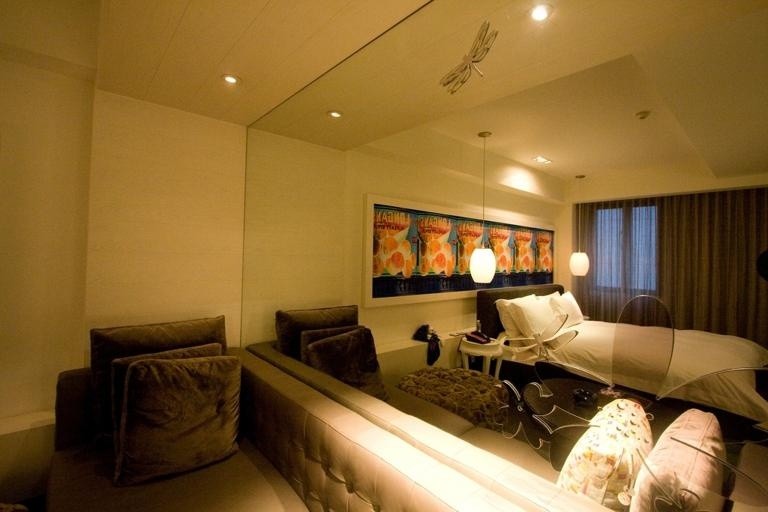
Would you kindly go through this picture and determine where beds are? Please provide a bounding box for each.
[477,284,768,435]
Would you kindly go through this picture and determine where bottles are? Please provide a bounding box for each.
[476,320,481,332]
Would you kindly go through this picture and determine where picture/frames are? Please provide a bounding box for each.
[362,193,557,308]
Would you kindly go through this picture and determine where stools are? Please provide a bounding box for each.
[459,337,503,380]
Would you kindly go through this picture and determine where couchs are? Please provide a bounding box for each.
[246,340,768,512]
[47,348,526,511]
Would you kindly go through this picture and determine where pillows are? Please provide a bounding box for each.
[112,344,221,459]
[116,357,241,483]
[553,399,653,512]
[537,290,560,309]
[507,294,556,337]
[550,292,584,326]
[90,315,224,452]
[626,408,726,511]
[300,325,366,366]
[493,294,537,336]
[275,305,359,363]
[308,328,389,402]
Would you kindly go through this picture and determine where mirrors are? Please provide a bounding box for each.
[240,1,767,512]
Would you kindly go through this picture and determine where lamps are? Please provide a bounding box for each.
[568,175,590,276]
[470,132,495,283]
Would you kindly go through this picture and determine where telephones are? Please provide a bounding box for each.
[465,331,490,343]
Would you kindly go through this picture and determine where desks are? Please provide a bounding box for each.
[524,378,652,472]
[396,367,510,432]
